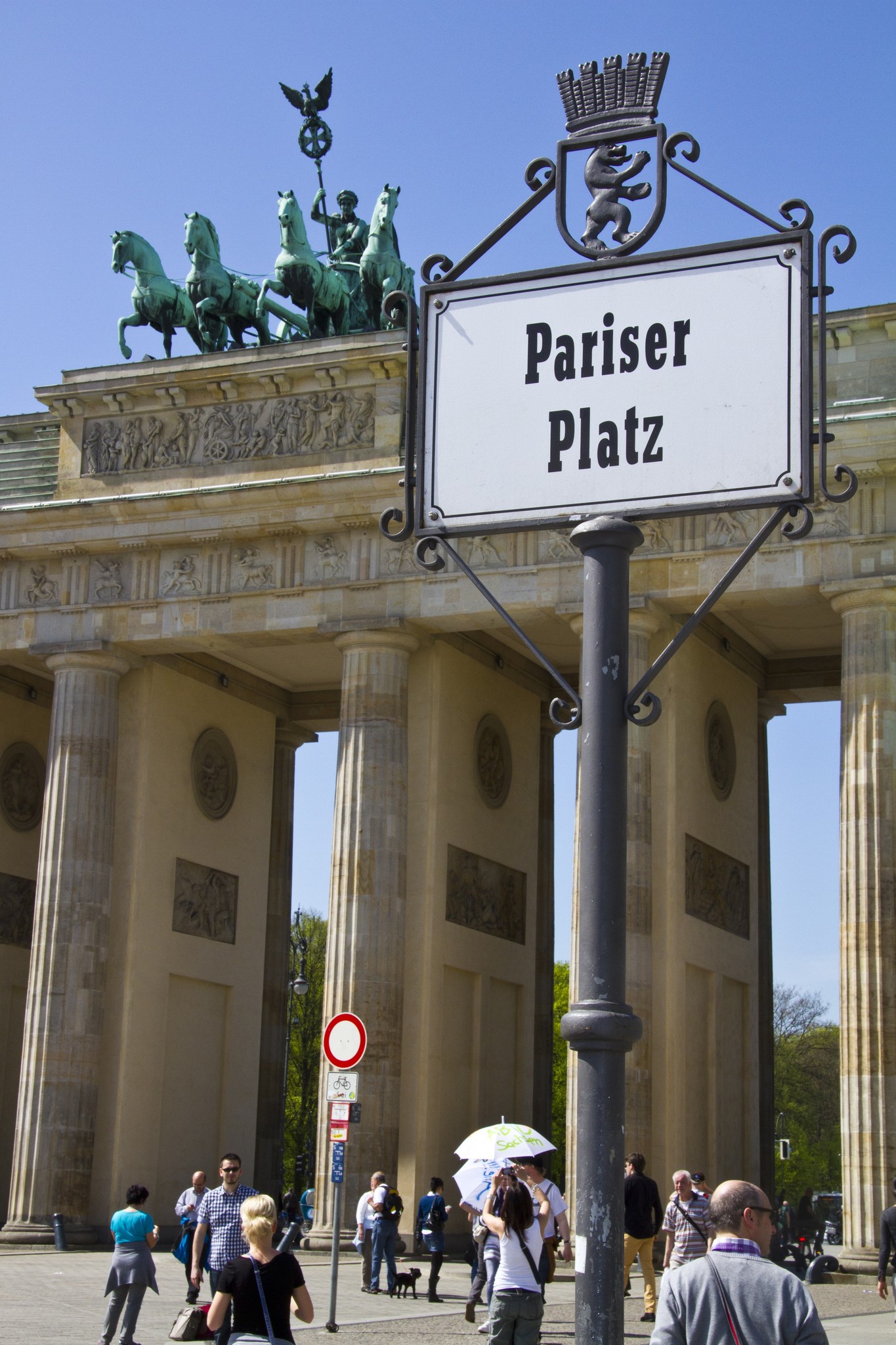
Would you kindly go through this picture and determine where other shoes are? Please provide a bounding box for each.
[624,1292,631,1298]
[186,1296,196,1304]
[476,1298,483,1304]
[118,1340,142,1345]
[465,1302,475,1323]
[97,1339,110,1345]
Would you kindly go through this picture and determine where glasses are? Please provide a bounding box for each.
[624,1164,630,1168]
[741,1206,776,1226]
[222,1167,240,1172]
[441,1187,444,1191]
[194,1182,205,1186]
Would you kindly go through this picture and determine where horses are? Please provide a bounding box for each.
[108,229,196,358]
[259,189,353,338]
[174,212,259,355]
[361,182,416,330]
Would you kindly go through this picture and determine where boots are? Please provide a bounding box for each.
[428,1275,444,1303]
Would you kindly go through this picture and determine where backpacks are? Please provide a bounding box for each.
[374,1186,404,1221]
[169,1306,207,1341]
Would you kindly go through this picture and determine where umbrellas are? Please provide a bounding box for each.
[453,1116,558,1192]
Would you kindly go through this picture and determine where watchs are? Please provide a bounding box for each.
[153,1233,159,1240]
[564,1240,572,1245]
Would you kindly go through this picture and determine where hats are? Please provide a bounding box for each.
[691,1171,705,1182]
[515,1154,542,1164]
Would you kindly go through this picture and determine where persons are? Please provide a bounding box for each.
[282,1188,299,1230]
[204,1195,315,1345]
[616,1149,824,1327]
[175,1170,213,1304]
[190,1152,265,1345]
[503,1156,573,1345]
[650,1180,829,1345]
[413,1175,451,1304]
[310,187,368,326]
[478,1170,522,1335]
[481,1164,550,1345]
[876,1178,896,1321]
[97,1185,160,1345]
[464,1180,571,1324]
[352,1171,404,1298]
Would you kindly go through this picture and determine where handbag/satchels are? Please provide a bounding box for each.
[463,1225,489,1268]
[193,1303,217,1338]
[425,1195,445,1232]
[538,1243,550,1305]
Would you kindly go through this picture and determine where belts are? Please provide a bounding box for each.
[373,1216,388,1223]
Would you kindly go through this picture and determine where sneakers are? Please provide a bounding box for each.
[367,1289,378,1294]
[361,1287,383,1293]
[477,1319,489,1334]
[640,1312,656,1322]
[381,1289,397,1295]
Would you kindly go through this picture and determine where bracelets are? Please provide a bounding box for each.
[531,1183,539,1192]
[485,1193,495,1201]
